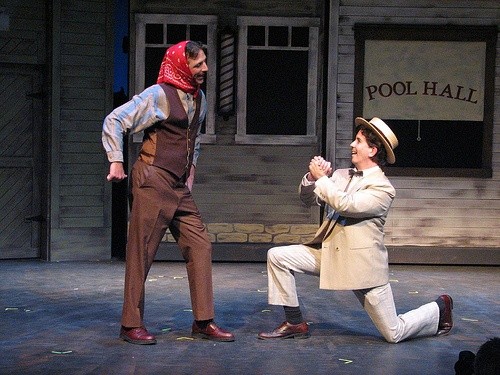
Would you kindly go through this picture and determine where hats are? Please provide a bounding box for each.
[355,117,398,164]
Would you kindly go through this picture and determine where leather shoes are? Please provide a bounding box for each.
[257,321,310,340]
[120,325,155,345]
[435,294,453,337]
[191,321,235,341]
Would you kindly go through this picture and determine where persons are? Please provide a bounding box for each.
[471,337,500,375]
[101,40,235,344]
[258,118,454,343]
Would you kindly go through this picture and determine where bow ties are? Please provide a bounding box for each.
[349,169,363,176]
